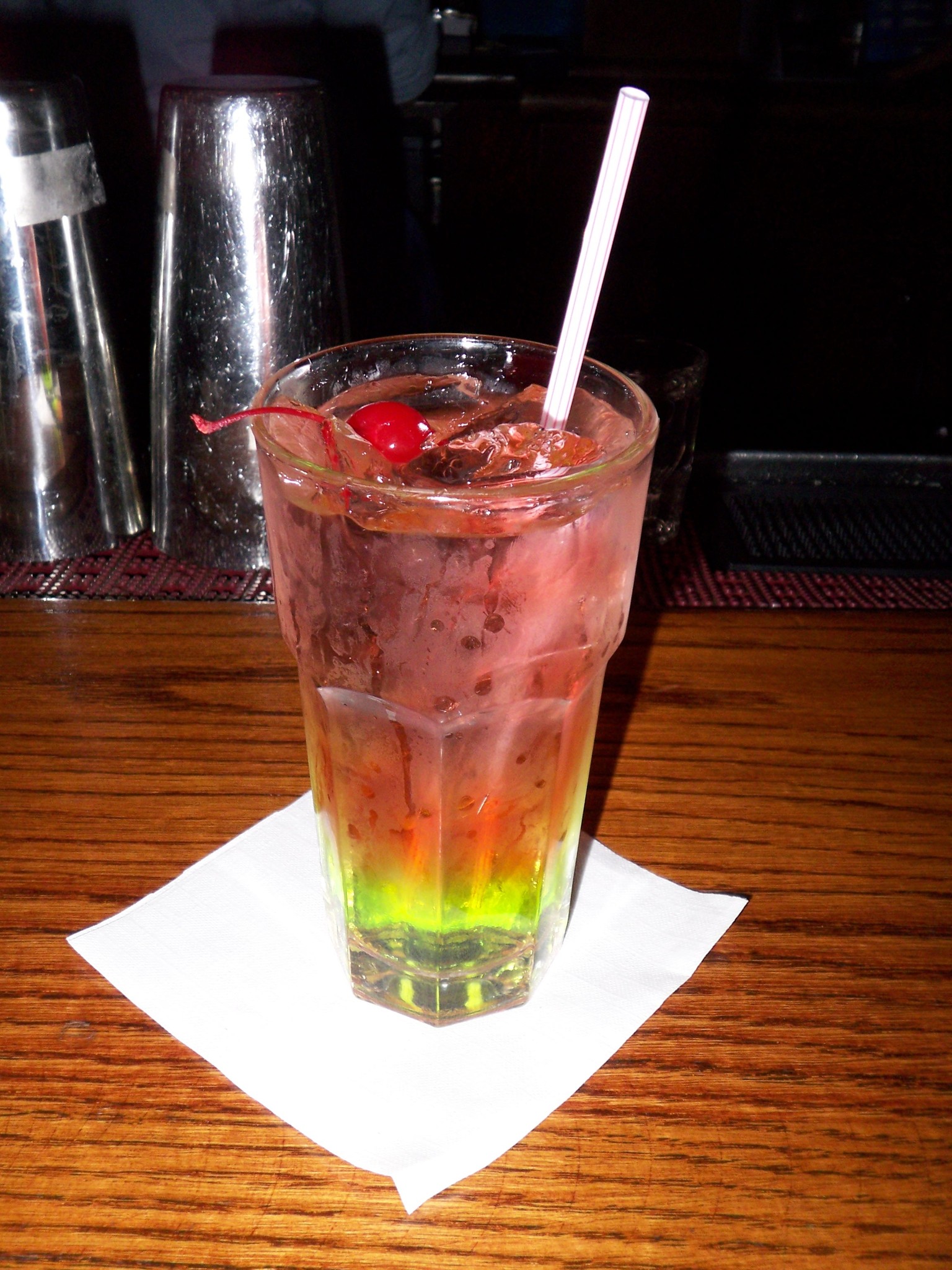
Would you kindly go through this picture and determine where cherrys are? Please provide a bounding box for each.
[192,403,432,468]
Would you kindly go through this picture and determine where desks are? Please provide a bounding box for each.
[0,452,952,1270]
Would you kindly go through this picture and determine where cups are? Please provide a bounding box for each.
[152,72,347,576]
[0,77,146,561]
[247,334,661,1029]
